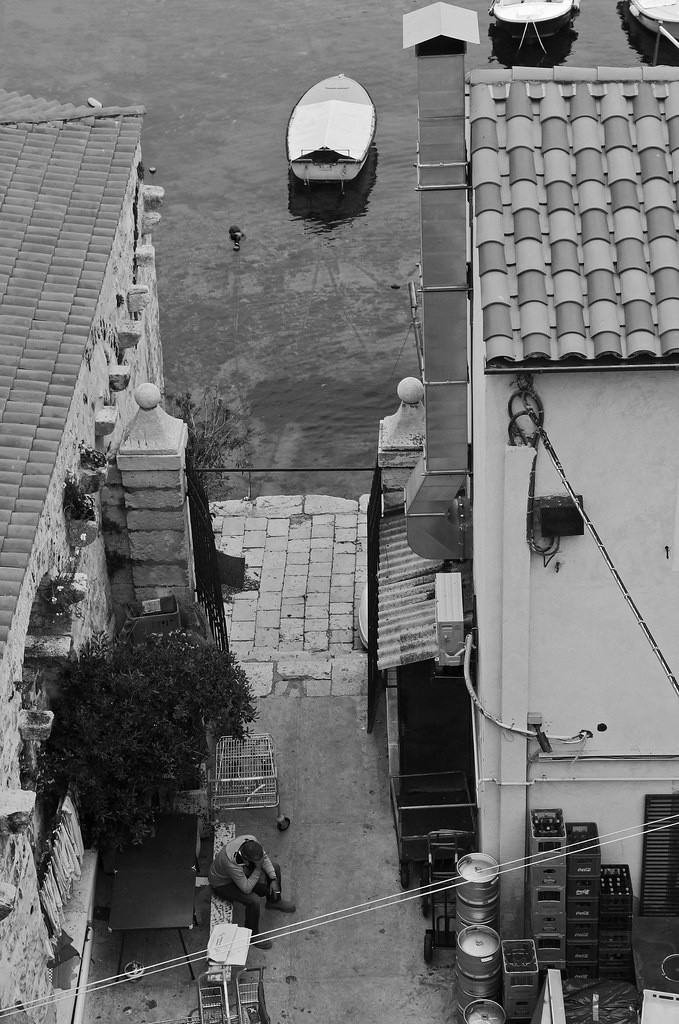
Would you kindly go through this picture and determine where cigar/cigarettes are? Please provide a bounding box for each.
[276,892,280,893]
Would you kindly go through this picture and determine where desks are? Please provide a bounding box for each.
[107,813,199,932]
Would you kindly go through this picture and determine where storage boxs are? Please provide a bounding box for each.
[124,593,181,646]
[501,808,634,1024]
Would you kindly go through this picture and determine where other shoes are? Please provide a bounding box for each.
[251,933,272,949]
[266,900,296,912]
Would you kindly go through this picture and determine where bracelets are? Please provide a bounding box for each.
[271,878,276,881]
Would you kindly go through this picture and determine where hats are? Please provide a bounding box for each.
[242,842,264,863]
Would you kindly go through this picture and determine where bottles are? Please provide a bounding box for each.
[568,830,598,854]
[600,867,630,904]
[532,809,565,852]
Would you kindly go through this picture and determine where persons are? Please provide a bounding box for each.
[208,835,296,949]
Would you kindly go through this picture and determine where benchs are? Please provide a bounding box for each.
[206,822,236,982]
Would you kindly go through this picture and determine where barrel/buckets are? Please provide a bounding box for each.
[456,852,507,1024]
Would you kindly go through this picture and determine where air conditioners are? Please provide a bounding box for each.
[435,572,466,668]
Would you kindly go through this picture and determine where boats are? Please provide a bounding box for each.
[488,0,581,39]
[285,72,378,196]
[286,137,379,232]
[629,0,679,42]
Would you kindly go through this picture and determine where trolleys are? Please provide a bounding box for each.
[209,732,290,832]
